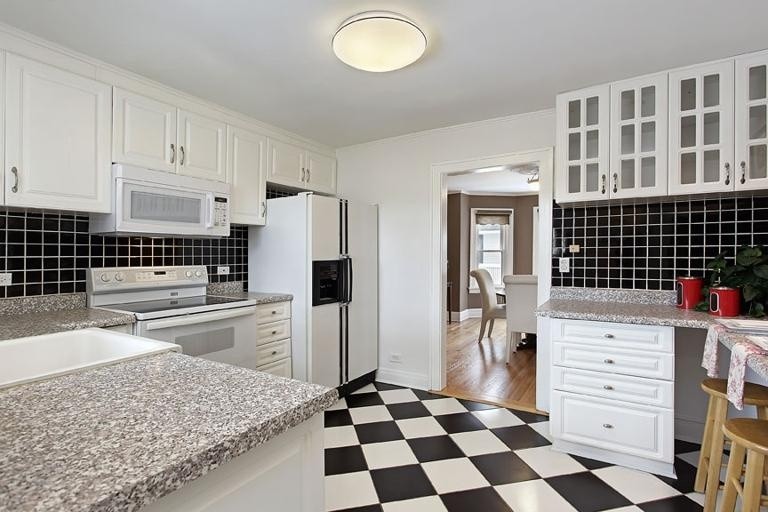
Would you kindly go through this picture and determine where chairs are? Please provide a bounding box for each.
[503,274,538,366]
[470,268,520,344]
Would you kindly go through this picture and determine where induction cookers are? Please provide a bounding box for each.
[94,293,249,314]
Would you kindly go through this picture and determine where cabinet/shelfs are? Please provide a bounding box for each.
[227,127,266,226]
[258,302,293,381]
[267,138,337,193]
[0,51,111,216]
[668,50,768,196]
[550,319,675,466]
[556,73,667,204]
[111,90,233,184]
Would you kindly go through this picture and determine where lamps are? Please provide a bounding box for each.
[332,11,427,74]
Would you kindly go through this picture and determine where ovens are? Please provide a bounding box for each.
[142,321,257,357]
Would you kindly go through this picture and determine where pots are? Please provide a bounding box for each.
[675,275,705,309]
[709,287,740,317]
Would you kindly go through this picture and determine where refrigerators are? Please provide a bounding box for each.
[306,192,378,380]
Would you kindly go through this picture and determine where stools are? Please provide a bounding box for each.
[694,378,768,512]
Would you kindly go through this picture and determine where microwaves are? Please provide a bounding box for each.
[115,179,232,238]
